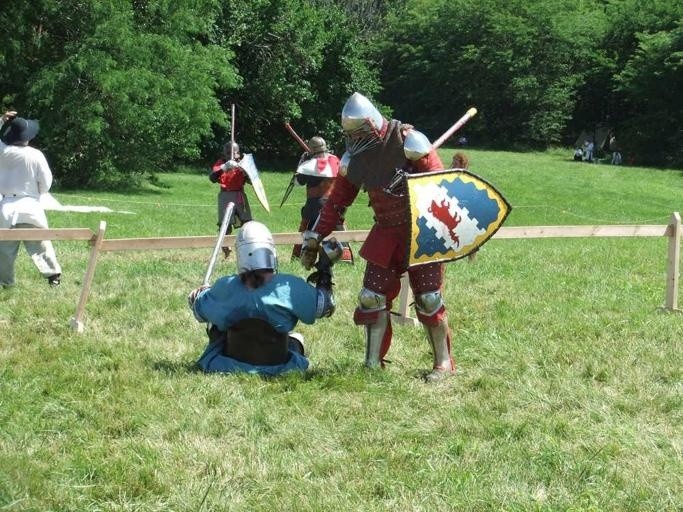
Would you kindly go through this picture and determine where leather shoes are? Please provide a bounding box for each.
[301,152,309,159]
[300,231,322,271]
[314,239,344,271]
[220,160,237,172]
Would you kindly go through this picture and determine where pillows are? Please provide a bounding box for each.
[222,141,240,161]
[309,136,329,154]
[235,221,278,275]
[341,92,383,157]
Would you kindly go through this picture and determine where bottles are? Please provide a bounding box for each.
[0,118,39,145]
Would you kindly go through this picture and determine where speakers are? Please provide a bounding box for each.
[415,299,455,381]
[354,303,392,368]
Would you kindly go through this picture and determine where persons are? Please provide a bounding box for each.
[304,92,458,385]
[573,128,623,165]
[209,139,259,260]
[188,219,333,377]
[293,137,352,267]
[0,109,65,293]
[438,151,488,265]
